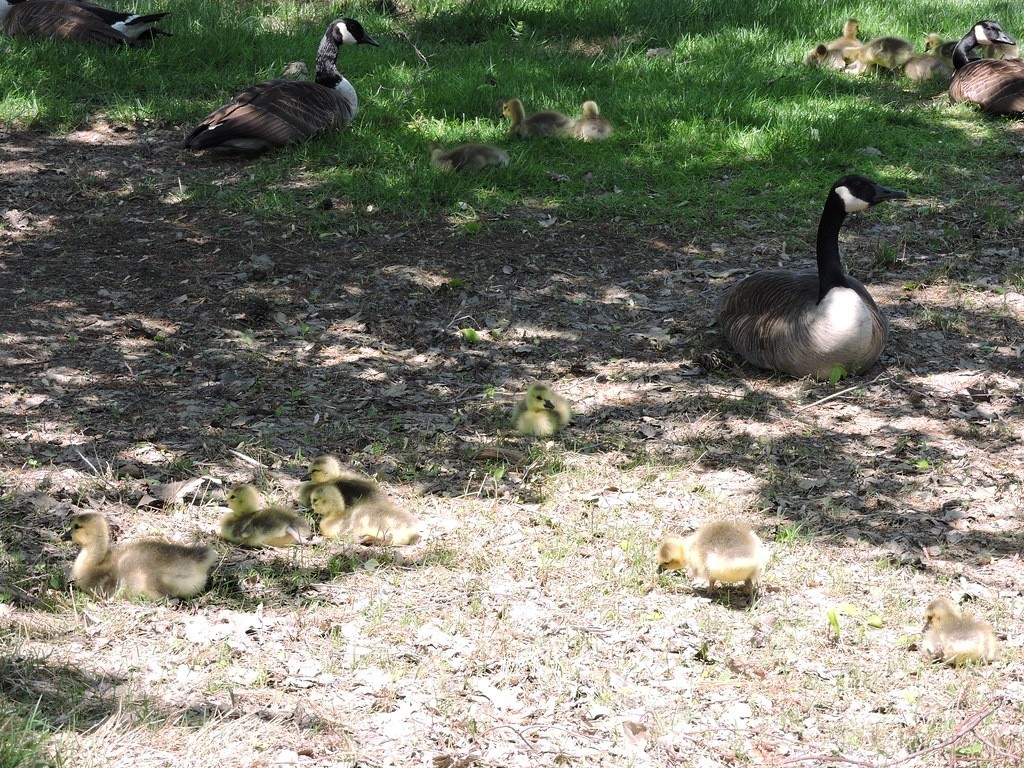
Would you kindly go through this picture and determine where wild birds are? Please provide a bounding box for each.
[218,484,324,548]
[428,98,614,177]
[0,0,175,52]
[655,519,769,599]
[60,509,217,603]
[919,597,999,666]
[179,17,381,156]
[510,385,572,438]
[716,173,909,383]
[299,456,429,546]
[801,19,1024,116]
